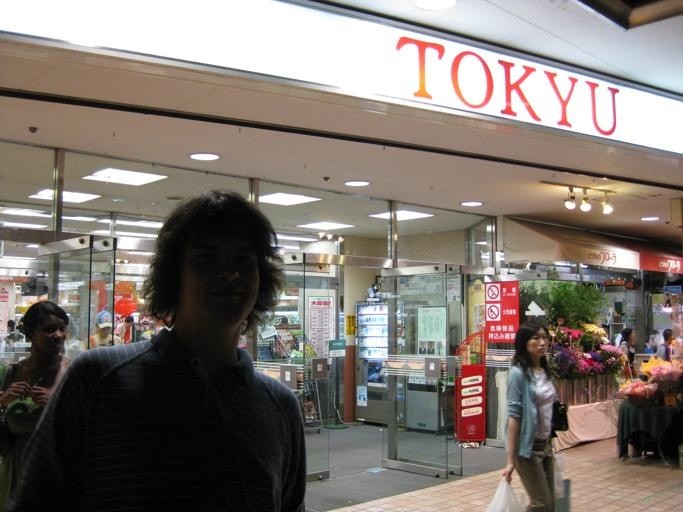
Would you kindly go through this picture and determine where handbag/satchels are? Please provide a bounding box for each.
[553,401,568,430]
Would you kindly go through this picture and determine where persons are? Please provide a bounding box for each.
[114,315,134,344]
[13,190,309,511]
[84,310,124,352]
[618,327,639,380]
[274,315,299,359]
[4,318,24,346]
[656,328,673,364]
[0,299,76,511]
[499,318,563,512]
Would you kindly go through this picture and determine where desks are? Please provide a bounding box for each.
[617,401,682,458]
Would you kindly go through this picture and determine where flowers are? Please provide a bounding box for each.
[546,319,682,400]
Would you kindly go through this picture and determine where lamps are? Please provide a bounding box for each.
[563,185,592,212]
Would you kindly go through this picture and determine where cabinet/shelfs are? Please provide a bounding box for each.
[354,299,428,428]
